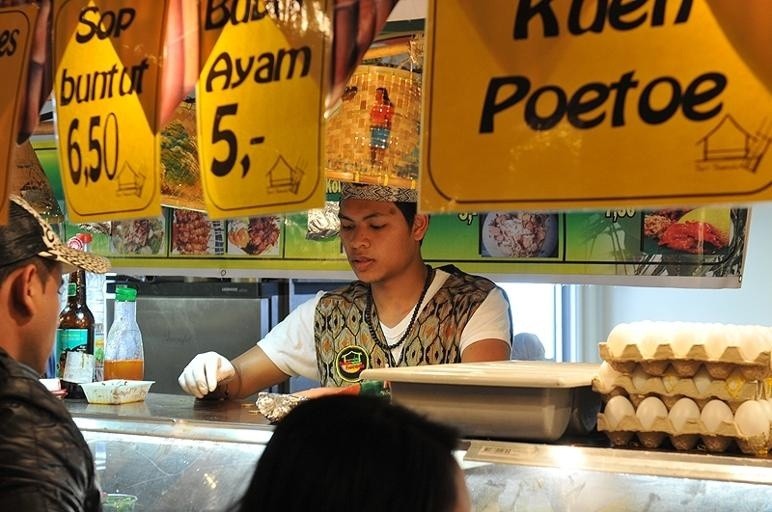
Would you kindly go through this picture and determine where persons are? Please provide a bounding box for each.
[0,190,111,512]
[223,394,472,512]
[176,180,512,403]
[370,88,396,166]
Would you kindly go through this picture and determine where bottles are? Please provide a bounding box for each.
[56,232,145,400]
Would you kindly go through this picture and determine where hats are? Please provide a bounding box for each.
[0,193,111,274]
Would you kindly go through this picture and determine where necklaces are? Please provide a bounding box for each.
[365,264,432,349]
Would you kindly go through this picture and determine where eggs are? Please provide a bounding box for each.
[597,322,772,434]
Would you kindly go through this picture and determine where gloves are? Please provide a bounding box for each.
[177,351,236,399]
